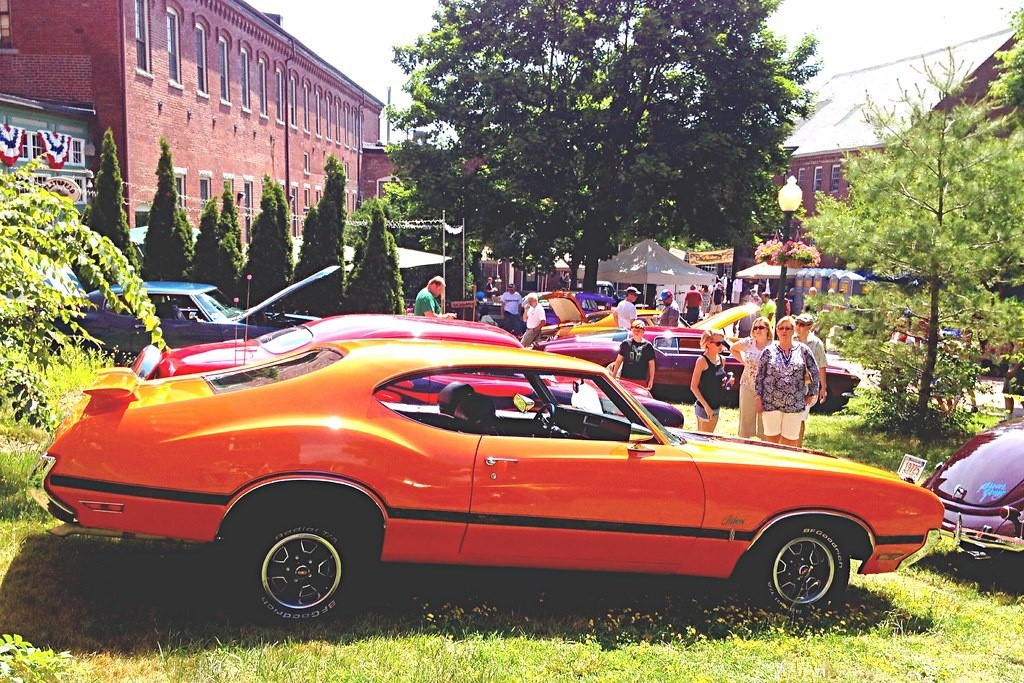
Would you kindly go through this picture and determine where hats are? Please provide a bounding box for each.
[632,320,645,329]
[507,283,515,289]
[750,289,756,292]
[627,286,641,294]
[797,313,813,322]
[658,289,672,300]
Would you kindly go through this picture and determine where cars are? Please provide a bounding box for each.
[920,415,1024,595]
[548,303,762,339]
[132,312,655,414]
[476,281,625,333]
[534,326,861,417]
[74,265,347,368]
[27,338,946,623]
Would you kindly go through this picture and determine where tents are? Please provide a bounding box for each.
[569,238,717,310]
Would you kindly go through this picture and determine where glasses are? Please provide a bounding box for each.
[796,321,811,327]
[709,341,724,345]
[754,326,767,330]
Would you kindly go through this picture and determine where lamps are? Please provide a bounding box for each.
[84,145,96,157]
[289,195,295,201]
[86,178,93,188]
[238,192,246,200]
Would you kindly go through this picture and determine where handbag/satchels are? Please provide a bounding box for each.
[711,304,717,312]
[801,346,815,404]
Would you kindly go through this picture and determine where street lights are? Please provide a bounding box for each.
[777,174,803,325]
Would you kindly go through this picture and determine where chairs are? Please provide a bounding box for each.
[452,392,503,432]
[438,381,480,413]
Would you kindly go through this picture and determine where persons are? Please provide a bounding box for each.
[699,273,731,318]
[690,329,735,433]
[479,307,498,327]
[889,309,1024,423]
[614,287,641,331]
[520,291,546,347]
[801,287,856,331]
[414,276,456,319]
[500,283,523,337]
[681,285,702,327]
[753,316,819,446]
[733,281,793,339]
[792,313,828,449]
[653,289,680,327]
[730,317,774,441]
[556,271,569,291]
[484,276,498,302]
[613,319,656,391]
